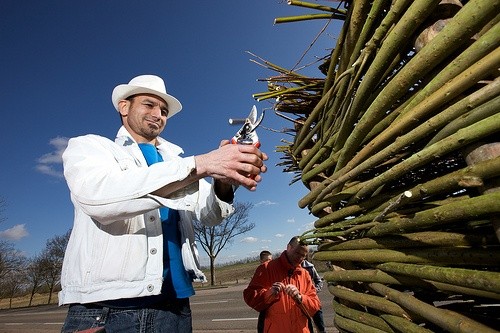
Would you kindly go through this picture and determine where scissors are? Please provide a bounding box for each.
[227,105,265,149]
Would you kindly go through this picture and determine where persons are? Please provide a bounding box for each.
[298,255,327,333]
[259,250,272,264]
[242,236,322,333]
[57,75,268,333]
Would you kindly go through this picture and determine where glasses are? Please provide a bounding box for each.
[288,242,306,260]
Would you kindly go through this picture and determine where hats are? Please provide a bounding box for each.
[111,74,183,120]
